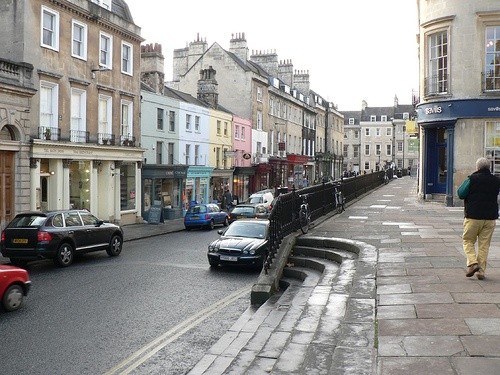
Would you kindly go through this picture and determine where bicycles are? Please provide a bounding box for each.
[298,193,314,234]
[382,166,389,185]
[331,182,346,214]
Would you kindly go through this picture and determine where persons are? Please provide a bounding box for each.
[225,190,233,211]
[457,157,500,280]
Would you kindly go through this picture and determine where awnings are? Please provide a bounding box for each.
[142,164,189,179]
[287,154,309,164]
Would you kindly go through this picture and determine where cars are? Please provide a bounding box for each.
[184,193,276,231]
[207,217,271,271]
[0,265,32,311]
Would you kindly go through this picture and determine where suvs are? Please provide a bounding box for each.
[1,209,124,267]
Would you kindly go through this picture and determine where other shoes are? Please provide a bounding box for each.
[466,263,485,280]
